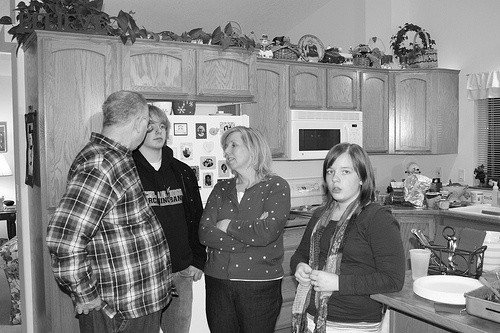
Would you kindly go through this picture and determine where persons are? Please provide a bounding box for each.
[205,175,211,186]
[133,102,208,333]
[289,142,406,333]
[220,163,227,174]
[203,159,213,167]
[45,90,179,333]
[197,127,204,137]
[197,127,291,333]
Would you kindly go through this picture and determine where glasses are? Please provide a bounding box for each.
[141,117,154,132]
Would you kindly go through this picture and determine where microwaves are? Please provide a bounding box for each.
[285,110,363,160]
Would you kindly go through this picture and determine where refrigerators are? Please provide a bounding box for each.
[159,115,249,333]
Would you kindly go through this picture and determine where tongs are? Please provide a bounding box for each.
[410,228,449,275]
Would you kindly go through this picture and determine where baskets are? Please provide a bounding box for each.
[222,20,242,46]
[271,44,309,62]
[380,54,392,66]
[354,57,371,67]
[406,30,438,68]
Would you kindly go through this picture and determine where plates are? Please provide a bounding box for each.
[298,34,325,62]
[413,275,484,305]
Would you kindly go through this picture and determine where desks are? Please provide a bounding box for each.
[0,204,17,239]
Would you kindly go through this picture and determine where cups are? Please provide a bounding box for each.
[471,192,484,203]
[439,199,449,210]
[409,249,431,281]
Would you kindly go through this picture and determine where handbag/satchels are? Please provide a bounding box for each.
[0,235,21,324]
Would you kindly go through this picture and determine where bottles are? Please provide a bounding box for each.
[492,182,498,207]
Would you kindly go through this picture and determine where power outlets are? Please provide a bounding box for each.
[436,167,443,179]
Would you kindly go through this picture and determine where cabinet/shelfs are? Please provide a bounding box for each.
[22,29,500,333]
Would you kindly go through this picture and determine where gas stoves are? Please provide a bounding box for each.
[284,176,326,226]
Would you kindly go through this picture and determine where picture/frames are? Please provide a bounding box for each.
[0,121,8,152]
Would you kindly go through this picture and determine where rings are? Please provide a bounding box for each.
[313,281,315,287]
